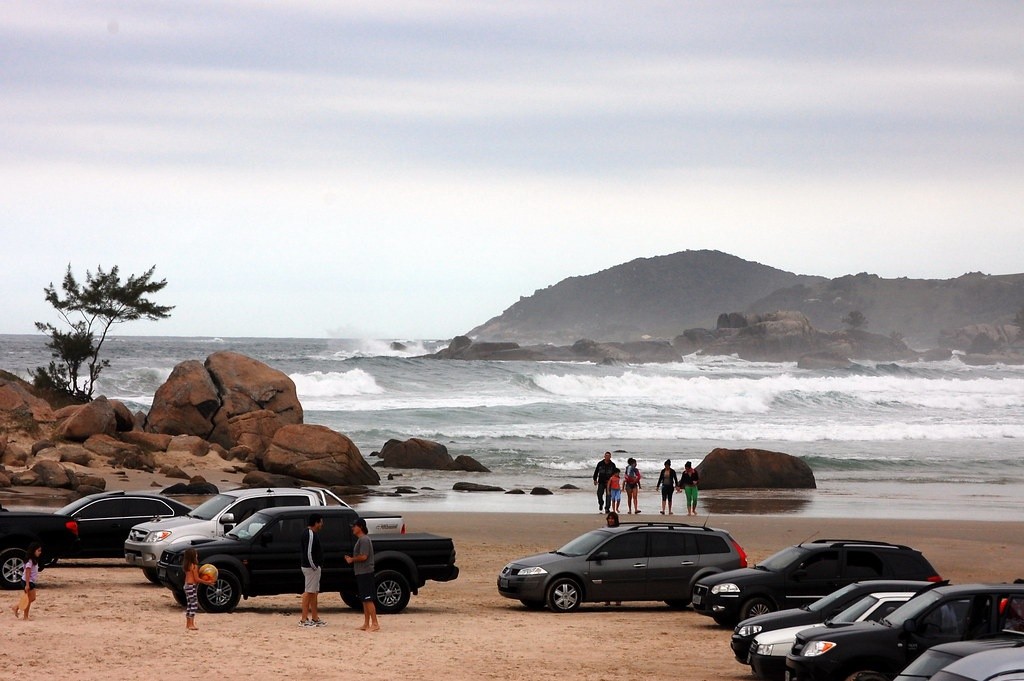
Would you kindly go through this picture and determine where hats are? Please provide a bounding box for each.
[349,518,366,526]
[664,459,671,466]
[685,461,691,467]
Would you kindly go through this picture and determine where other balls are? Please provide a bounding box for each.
[199,564,219,584]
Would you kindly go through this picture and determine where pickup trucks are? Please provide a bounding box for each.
[156,506,459,614]
[125,487,406,588]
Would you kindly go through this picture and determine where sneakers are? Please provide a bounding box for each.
[311,618,328,626]
[298,619,314,628]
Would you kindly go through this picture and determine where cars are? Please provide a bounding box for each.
[731,580,1024,681]
[0,503,80,590]
[43,491,194,568]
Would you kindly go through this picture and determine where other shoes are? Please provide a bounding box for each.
[604,601,610,608]
[660,511,665,515]
[635,510,641,514]
[599,501,604,511]
[616,601,621,607]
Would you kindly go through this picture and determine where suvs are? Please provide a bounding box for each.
[497,521,748,613]
[693,538,943,628]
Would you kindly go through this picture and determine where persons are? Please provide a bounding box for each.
[656,459,680,515]
[927,574,960,636]
[298,516,328,629]
[679,461,698,516]
[607,468,621,513]
[622,457,643,515]
[603,513,623,606]
[10,546,43,623]
[182,547,215,630]
[345,519,379,633]
[593,453,616,513]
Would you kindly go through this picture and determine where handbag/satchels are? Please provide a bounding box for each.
[19,590,29,610]
